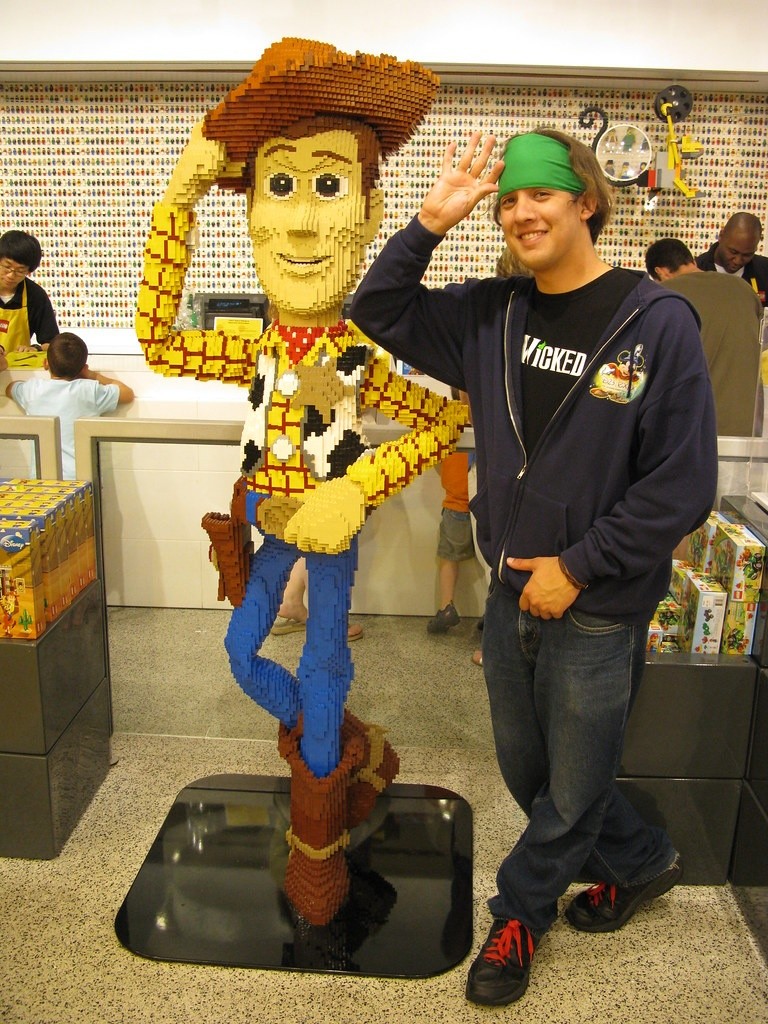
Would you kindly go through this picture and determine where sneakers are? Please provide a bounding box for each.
[465,918,540,1006]
[566,860,684,933]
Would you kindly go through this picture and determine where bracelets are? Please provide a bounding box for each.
[556,556,589,590]
[31,344,43,351]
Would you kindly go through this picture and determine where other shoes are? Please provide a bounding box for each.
[477,616,484,630]
[428,600,459,633]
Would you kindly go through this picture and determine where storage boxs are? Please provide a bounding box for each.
[721,596,758,655]
[649,621,662,653]
[661,641,679,653]
[688,511,730,573]
[0,494,79,600]
[0,521,45,640]
[669,558,699,605]
[655,594,680,634]
[710,524,765,603]
[0,507,59,623]
[0,484,87,592]
[0,500,71,613]
[0,478,97,582]
[677,572,728,655]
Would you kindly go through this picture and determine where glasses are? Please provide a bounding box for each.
[0,263,29,276]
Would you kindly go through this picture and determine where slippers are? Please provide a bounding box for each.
[348,624,363,641]
[271,618,306,635]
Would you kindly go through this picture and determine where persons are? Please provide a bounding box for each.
[428,385,494,666]
[140,32,474,939]
[646,239,765,439]
[270,550,365,645]
[5,332,134,483]
[349,125,717,1008]
[698,212,767,311]
[0,230,60,354]
